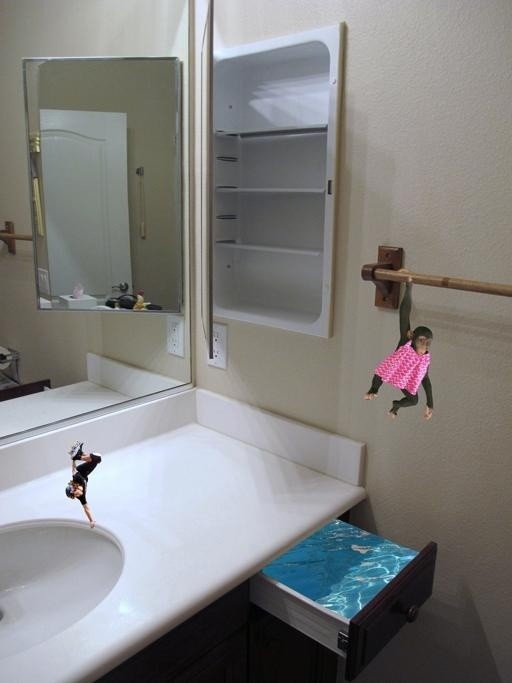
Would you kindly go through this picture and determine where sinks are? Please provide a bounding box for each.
[1,517,125,661]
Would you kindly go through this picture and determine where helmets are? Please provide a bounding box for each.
[66,486,75,499]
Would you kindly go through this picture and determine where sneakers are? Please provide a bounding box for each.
[72,442,84,460]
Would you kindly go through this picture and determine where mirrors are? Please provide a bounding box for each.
[1,56,193,439]
[20,57,185,313]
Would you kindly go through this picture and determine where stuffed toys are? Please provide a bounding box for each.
[362,268,434,420]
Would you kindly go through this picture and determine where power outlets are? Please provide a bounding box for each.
[207,322,228,371]
[165,316,185,359]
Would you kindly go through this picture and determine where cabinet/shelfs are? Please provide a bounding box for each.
[211,21,346,339]
[94,508,438,682]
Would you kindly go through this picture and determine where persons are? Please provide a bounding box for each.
[64,440,103,527]
